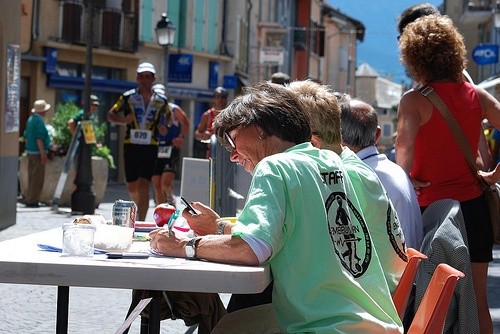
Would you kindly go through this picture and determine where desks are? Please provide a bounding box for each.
[0,220,271,334]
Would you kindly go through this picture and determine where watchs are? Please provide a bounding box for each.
[216,222,227,235]
[186,237,201,261]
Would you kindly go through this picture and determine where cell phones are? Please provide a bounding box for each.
[104,253,150,258]
[181,196,198,215]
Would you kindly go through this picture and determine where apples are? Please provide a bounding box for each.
[153,203,176,226]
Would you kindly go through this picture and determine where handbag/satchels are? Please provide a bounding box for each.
[487,183,500,245]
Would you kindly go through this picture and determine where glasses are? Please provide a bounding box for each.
[224,123,248,149]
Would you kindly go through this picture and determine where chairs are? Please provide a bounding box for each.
[393,248,465,334]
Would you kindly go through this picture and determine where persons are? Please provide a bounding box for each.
[226,78,409,314]
[22,99,51,209]
[107,63,190,221]
[395,4,500,334]
[339,99,424,252]
[148,82,403,334]
[195,87,229,158]
[67,94,100,191]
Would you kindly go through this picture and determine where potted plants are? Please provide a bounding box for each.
[19,100,117,208]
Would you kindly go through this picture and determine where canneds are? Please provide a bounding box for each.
[112,200,137,237]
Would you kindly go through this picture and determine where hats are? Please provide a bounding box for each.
[137,62,156,75]
[152,83,166,95]
[31,99,50,113]
[90,95,100,105]
[267,73,291,85]
[213,87,230,95]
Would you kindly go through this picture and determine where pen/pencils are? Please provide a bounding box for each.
[167,209,180,229]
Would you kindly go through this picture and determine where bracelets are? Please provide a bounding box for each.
[200,132,204,141]
[178,135,184,139]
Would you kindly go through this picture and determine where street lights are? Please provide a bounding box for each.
[153,11,177,98]
